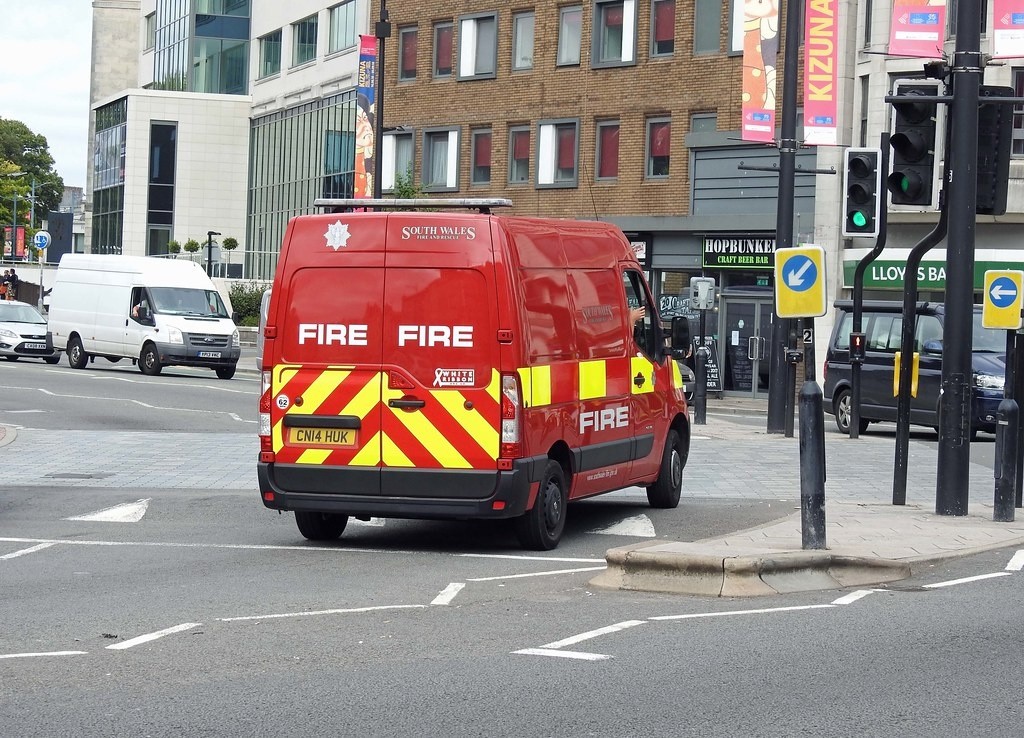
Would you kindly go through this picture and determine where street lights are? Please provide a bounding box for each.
[206,231,221,281]
[0,190,38,265]
[30,179,52,261]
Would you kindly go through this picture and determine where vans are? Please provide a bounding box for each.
[256,289,272,370]
[257,196,693,547]
[823,301,1005,442]
[46,252,241,380]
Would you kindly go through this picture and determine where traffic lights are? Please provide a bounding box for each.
[849,332,867,364]
[841,148,881,238]
[886,80,943,214]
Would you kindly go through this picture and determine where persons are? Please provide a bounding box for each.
[628,306,646,339]
[0,269,18,299]
[132,291,163,318]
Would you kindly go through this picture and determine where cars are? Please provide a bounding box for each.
[43,290,52,312]
[677,361,695,407]
[0,300,62,364]
